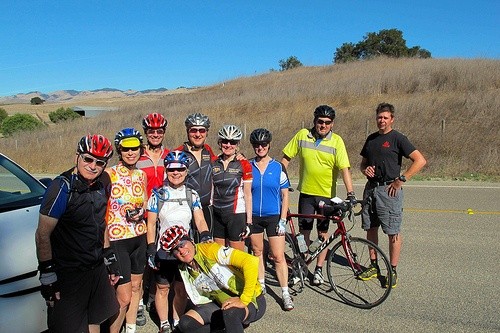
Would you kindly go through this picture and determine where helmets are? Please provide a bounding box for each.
[114,127,143,148]
[314,105,335,122]
[160,225,188,253]
[250,128,272,144]
[217,125,243,144]
[164,150,189,169]
[185,113,210,131]
[77,134,113,161]
[142,112,168,131]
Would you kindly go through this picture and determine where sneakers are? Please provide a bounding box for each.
[385,270,398,288]
[160,323,172,333]
[313,270,324,285]
[136,305,146,325]
[358,267,381,280]
[282,294,293,310]
[262,285,266,294]
[172,322,175,331]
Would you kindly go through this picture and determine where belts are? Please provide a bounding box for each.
[367,180,393,187]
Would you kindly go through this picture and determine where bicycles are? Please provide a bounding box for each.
[248,188,393,309]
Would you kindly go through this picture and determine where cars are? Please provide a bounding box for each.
[0,153,54,333]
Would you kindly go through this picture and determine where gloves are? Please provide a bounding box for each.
[345,196,358,207]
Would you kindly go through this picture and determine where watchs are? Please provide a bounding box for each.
[347,191,355,196]
[399,175,407,182]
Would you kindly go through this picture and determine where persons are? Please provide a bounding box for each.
[359,102,426,288]
[173,112,247,243]
[248,128,295,311]
[146,150,213,333]
[160,225,266,333]
[136,112,171,326]
[210,124,253,252]
[35,134,121,333]
[281,105,357,284]
[103,127,148,333]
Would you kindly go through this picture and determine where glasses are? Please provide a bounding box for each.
[221,139,239,145]
[167,168,186,172]
[119,147,139,152]
[80,154,106,167]
[253,142,268,147]
[147,129,165,134]
[189,128,208,133]
[316,119,332,125]
[172,240,186,254]
